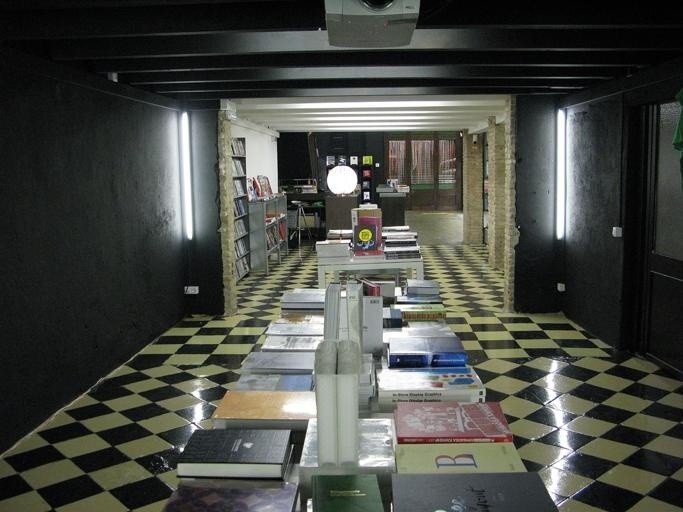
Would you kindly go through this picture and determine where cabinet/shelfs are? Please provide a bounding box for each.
[249,194,288,275]
[231,137,250,283]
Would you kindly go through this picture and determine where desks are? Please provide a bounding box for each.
[377,184,410,226]
[318,255,424,289]
[286,191,361,239]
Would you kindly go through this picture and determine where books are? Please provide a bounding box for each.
[230,140,250,284]
[247,175,274,200]
[375,178,409,193]
[315,202,421,261]
[265,213,287,250]
[176,276,560,511]
